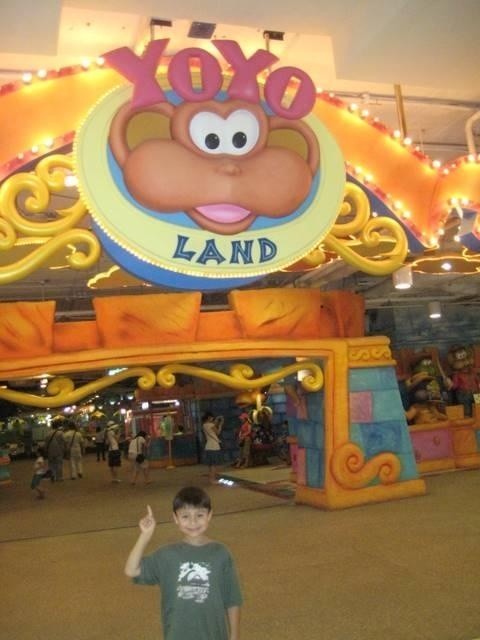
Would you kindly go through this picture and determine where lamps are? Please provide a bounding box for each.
[392,262,414,292]
[424,298,444,320]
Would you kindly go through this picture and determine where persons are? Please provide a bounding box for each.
[0,404,292,499]
[125,486,243,640]
